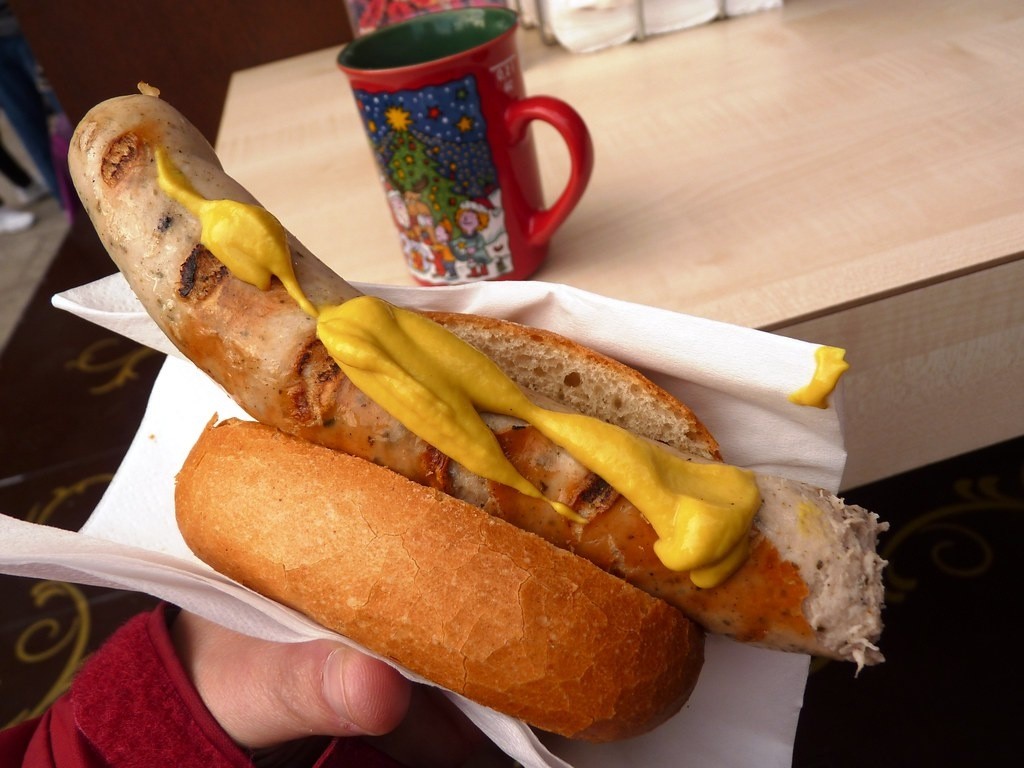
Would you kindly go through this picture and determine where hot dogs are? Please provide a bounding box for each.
[68,94,889,747]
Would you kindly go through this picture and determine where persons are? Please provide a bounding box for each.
[17,605,404,768]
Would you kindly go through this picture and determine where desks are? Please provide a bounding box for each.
[210,3,1024,498]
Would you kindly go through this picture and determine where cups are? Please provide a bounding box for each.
[335,6,594,286]
